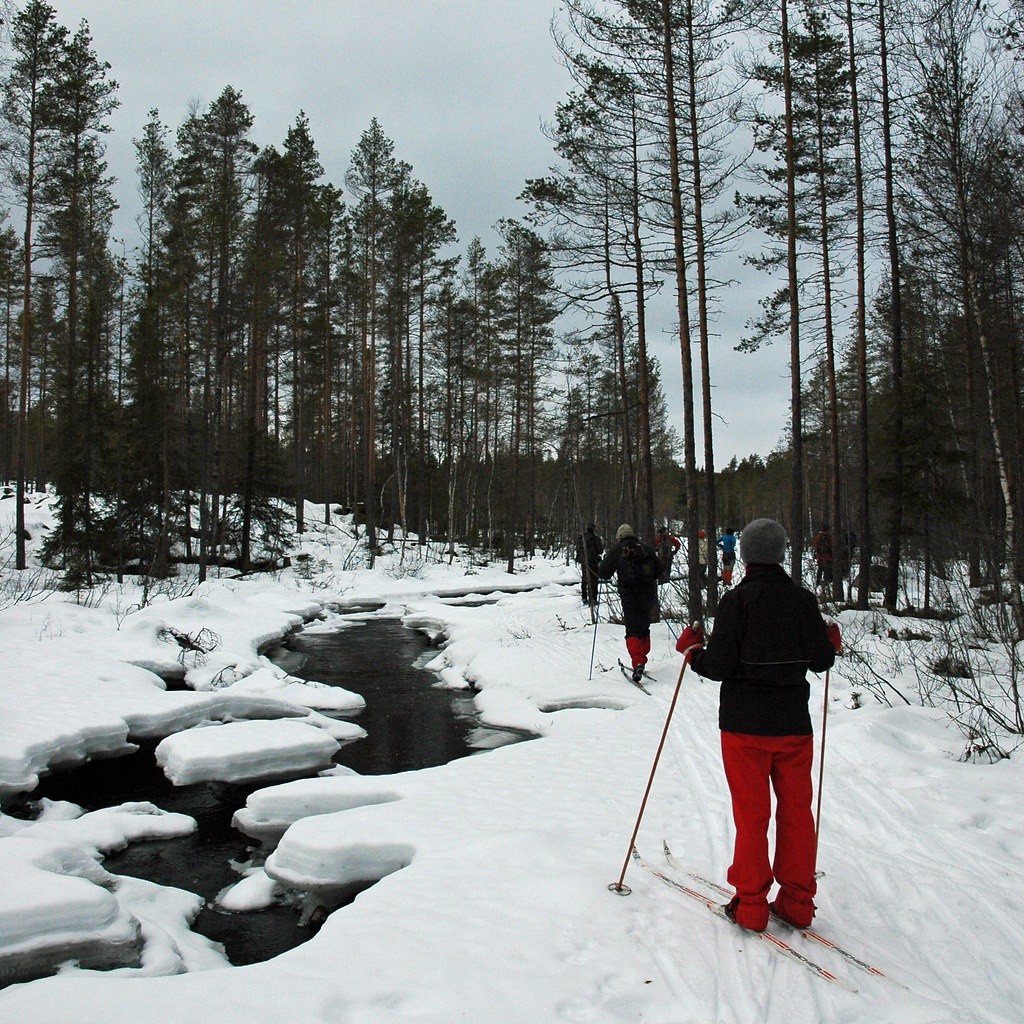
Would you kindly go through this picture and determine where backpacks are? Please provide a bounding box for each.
[659,534,672,559]
[617,541,656,589]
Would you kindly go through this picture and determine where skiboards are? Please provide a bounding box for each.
[618,658,657,696]
[629,839,914,995]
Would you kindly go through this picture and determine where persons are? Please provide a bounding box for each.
[655,526,681,585]
[575,522,603,606]
[698,528,708,588]
[813,525,832,585]
[598,524,663,682]
[716,528,736,586]
[675,518,841,933]
[843,525,857,579]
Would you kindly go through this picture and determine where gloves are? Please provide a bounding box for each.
[676,623,705,656]
[826,624,841,651]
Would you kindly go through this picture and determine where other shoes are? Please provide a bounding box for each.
[633,664,645,682]
[723,582,732,586]
[725,896,740,922]
[769,902,777,915]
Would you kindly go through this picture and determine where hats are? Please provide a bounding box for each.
[698,529,704,537]
[740,518,786,563]
[616,524,633,539]
